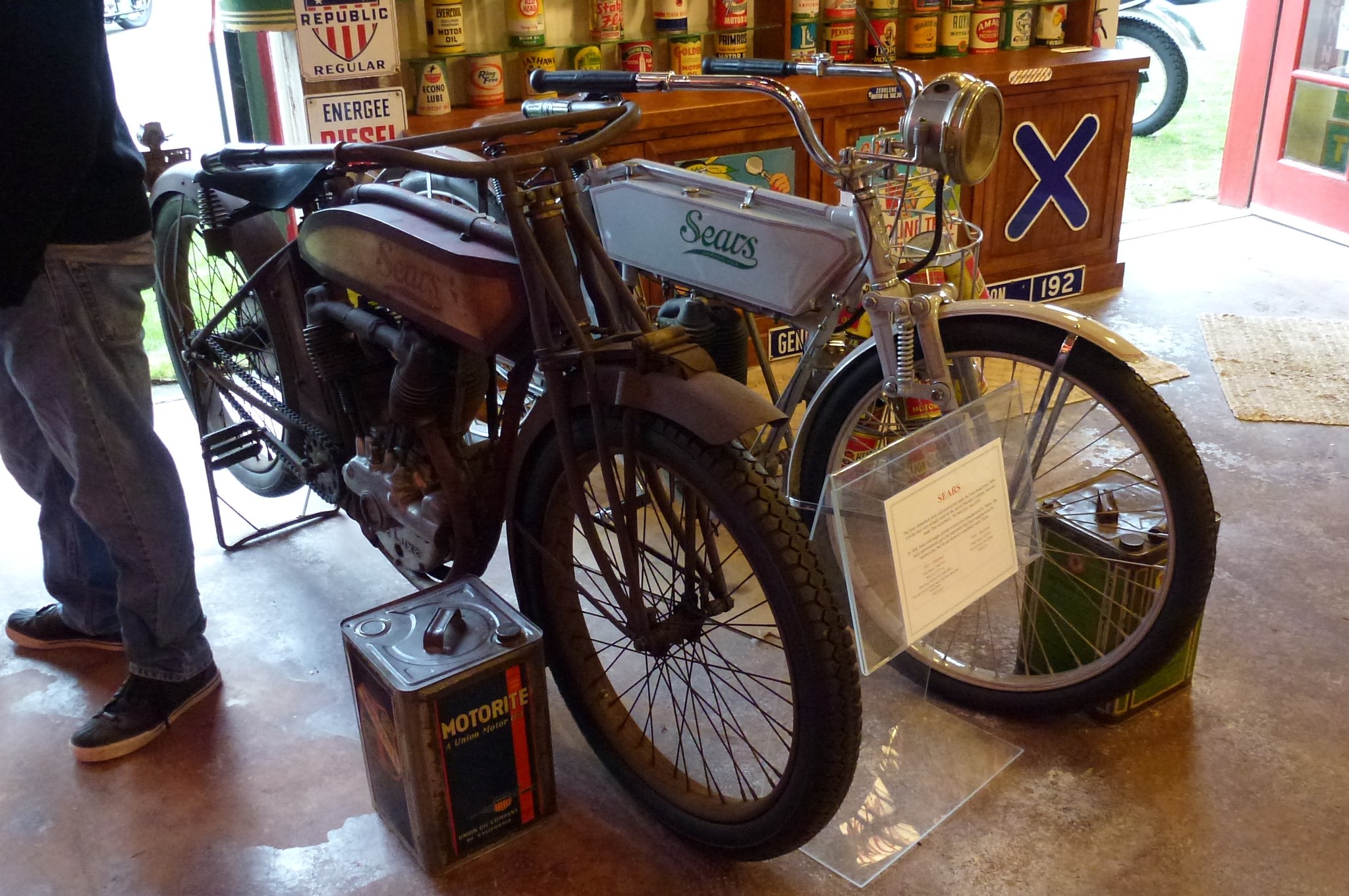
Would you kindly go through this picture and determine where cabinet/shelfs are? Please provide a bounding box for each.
[473,68,1135,416]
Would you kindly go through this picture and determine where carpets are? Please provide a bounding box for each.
[1198,310,1349,429]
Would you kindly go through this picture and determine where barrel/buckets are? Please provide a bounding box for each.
[407,0,747,119]
[1015,468,1221,725]
[340,575,561,882]
[781,0,1072,65]
[820,226,992,499]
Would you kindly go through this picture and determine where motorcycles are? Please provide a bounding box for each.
[1113,0,1207,138]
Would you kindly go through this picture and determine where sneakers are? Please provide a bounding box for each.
[4,602,122,652]
[69,660,222,762]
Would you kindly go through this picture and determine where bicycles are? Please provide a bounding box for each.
[148,51,1223,868]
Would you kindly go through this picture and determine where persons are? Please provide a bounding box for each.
[1,1,225,762]
